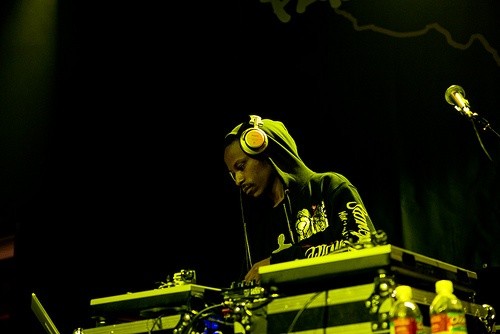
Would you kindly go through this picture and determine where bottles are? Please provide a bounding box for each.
[389,286,423,334]
[430,280,467,334]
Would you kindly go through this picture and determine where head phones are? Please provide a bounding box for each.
[239,114,268,156]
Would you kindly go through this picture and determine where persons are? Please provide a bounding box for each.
[223,115,377,285]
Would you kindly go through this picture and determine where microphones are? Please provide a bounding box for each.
[444,85,473,118]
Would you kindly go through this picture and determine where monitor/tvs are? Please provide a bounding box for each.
[31,292,60,334]
